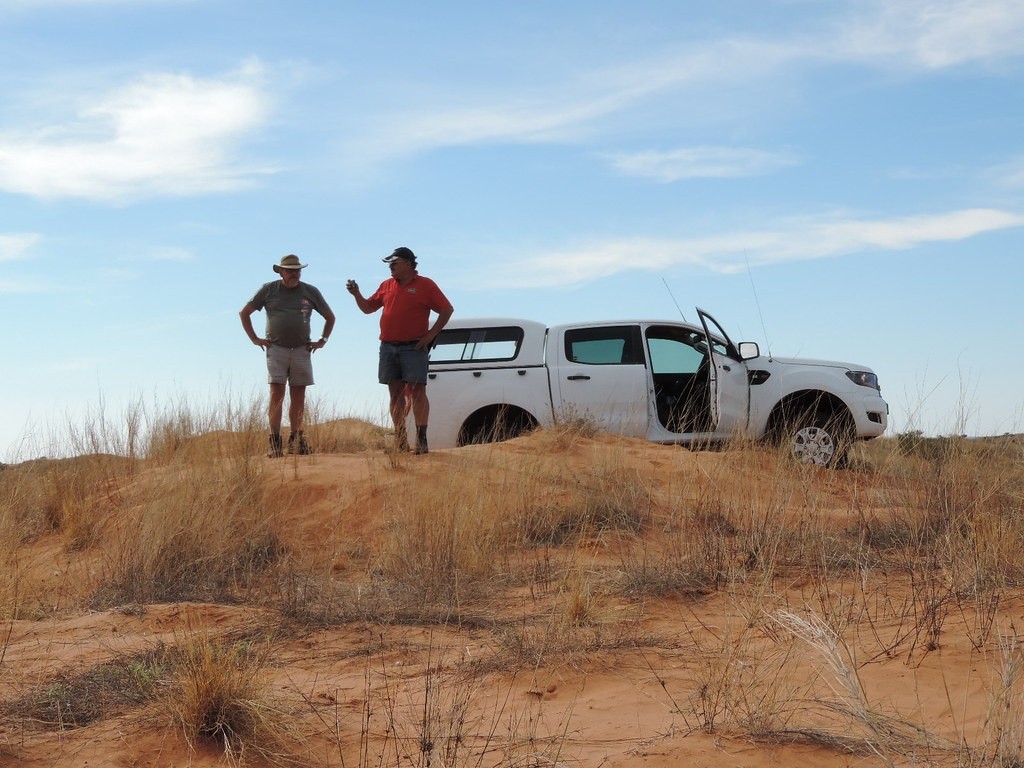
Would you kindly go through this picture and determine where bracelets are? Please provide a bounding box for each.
[319,336,328,343]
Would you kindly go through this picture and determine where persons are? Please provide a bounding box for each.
[347,247,453,455]
[239,254,335,456]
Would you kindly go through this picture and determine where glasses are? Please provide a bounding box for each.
[280,267,301,274]
[390,260,405,266]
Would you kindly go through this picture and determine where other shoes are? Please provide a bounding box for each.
[267,435,283,458]
[289,433,304,455]
[395,433,408,454]
[415,439,428,454]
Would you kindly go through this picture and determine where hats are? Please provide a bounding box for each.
[273,255,308,273]
[383,247,415,262]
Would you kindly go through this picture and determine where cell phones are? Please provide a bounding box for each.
[348,280,356,287]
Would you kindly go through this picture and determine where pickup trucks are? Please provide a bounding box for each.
[403,308,890,469]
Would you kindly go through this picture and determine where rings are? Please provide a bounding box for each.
[310,345,313,348]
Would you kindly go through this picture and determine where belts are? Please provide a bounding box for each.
[381,340,420,347]
